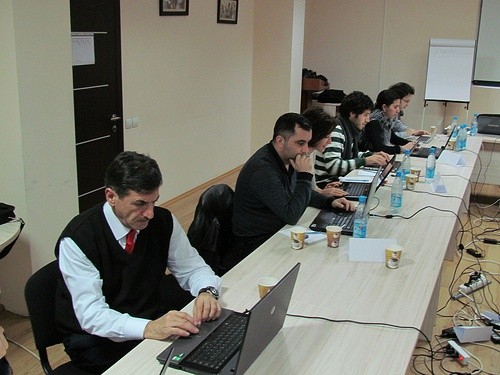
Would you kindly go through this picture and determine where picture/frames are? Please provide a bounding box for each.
[217,0,238,24]
[159,0,189,16]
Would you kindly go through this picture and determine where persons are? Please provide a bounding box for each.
[54,151,223,374]
[233,80,431,264]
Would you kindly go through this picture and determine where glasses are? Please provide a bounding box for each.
[402,98,410,103]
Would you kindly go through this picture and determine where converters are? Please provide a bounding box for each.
[442,324,463,338]
[466,249,481,258]
[484,238,497,244]
[489,320,500,330]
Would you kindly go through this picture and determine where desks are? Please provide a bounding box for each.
[100,133,500,375]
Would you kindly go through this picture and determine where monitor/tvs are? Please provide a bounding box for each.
[159,347,174,375]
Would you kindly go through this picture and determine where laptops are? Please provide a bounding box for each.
[309,165,382,235]
[364,139,419,172]
[333,162,394,201]
[409,124,456,159]
[415,118,444,143]
[156,262,301,375]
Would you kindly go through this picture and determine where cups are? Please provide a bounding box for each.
[449,140,456,150]
[405,174,418,190]
[259,276,280,301]
[430,125,436,136]
[446,128,451,137]
[385,244,402,268]
[326,225,343,248]
[291,226,306,250]
[411,167,421,181]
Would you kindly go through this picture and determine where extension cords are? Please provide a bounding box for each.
[458,274,487,294]
[447,341,470,365]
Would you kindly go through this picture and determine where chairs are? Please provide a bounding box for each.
[187,184,235,278]
[24,259,73,375]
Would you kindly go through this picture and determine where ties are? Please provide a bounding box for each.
[125,229,136,255]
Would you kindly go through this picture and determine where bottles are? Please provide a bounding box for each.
[391,171,402,215]
[353,196,368,238]
[463,124,467,149]
[454,124,465,152]
[425,148,436,183]
[470,113,477,136]
[450,116,459,139]
[402,149,411,186]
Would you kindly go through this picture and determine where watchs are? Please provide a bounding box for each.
[198,286,220,300]
